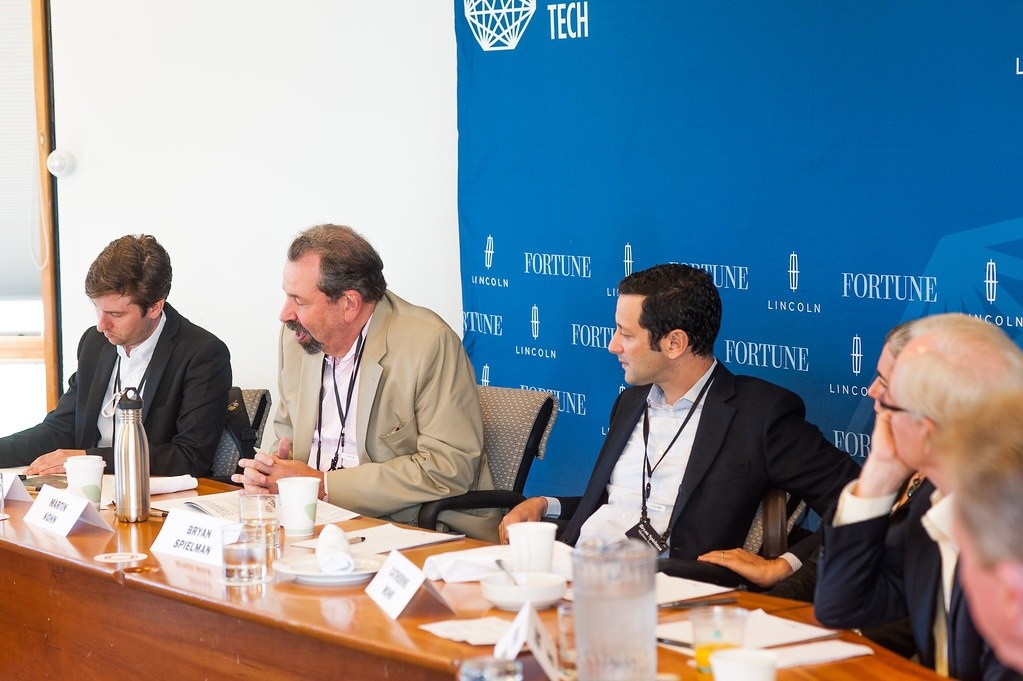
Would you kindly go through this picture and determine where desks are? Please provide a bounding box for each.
[0,476,959,681]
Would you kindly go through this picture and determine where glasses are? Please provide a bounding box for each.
[879,389,909,412]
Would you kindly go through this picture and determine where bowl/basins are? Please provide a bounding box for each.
[480,571,566,612]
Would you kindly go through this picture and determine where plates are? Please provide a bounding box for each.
[271,554,388,584]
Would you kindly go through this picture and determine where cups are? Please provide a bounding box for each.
[0,473,4,518]
[276,475,321,537]
[221,523,266,586]
[708,648,778,681]
[240,495,282,549]
[685,607,748,675]
[569,540,657,681]
[63,455,107,511]
[505,521,558,574]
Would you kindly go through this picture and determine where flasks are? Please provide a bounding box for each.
[101,387,151,523]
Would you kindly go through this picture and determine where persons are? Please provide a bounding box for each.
[230,225,502,545]
[815,315,1023,681]
[0,234,232,478]
[500,264,862,593]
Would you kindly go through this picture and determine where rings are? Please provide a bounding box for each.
[722,552,725,557]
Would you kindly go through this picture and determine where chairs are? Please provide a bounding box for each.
[737,486,809,561]
[210,386,274,481]
[415,384,559,531]
